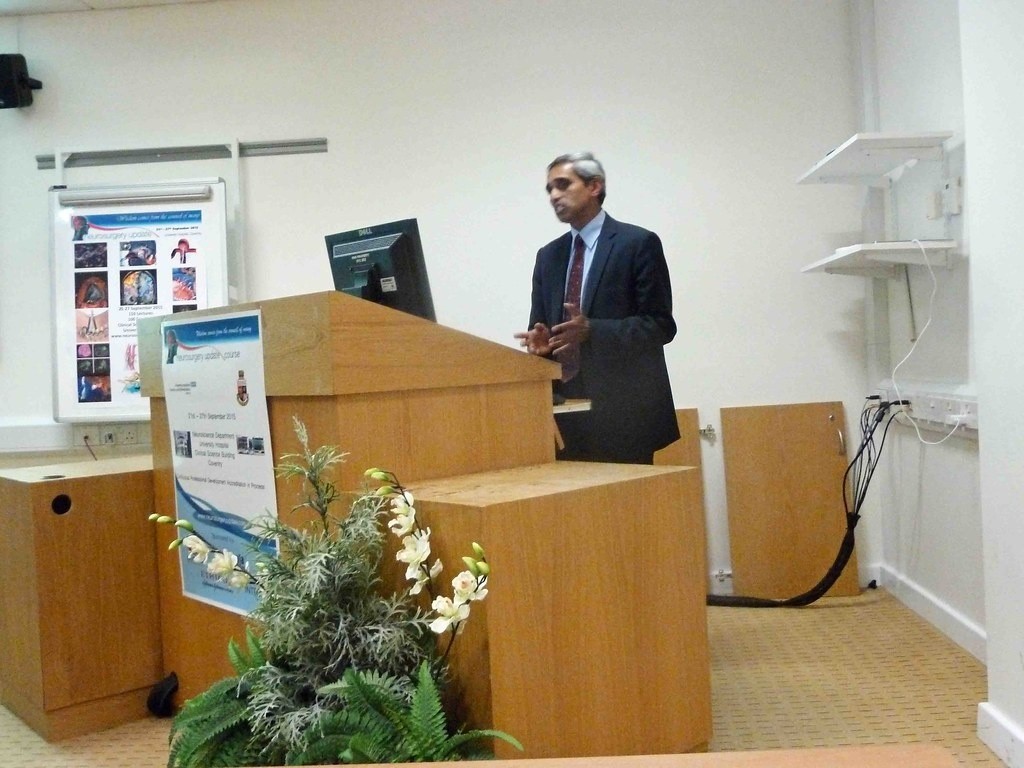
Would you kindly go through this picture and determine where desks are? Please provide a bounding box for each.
[382,459,712,760]
[0,453,169,745]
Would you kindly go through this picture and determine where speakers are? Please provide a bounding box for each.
[0,54,33,109]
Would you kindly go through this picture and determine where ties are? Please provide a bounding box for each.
[558,234,586,382]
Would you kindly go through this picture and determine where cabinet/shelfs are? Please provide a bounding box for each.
[798,129,958,279]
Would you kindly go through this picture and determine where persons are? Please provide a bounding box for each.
[514,152,680,465]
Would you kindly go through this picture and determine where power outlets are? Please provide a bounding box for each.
[99,425,118,444]
[73,424,99,446]
[870,389,978,430]
[118,424,136,445]
[137,424,151,444]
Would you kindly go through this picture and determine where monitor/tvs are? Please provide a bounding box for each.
[325,218,437,324]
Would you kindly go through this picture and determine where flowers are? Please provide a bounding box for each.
[147,411,522,767]
